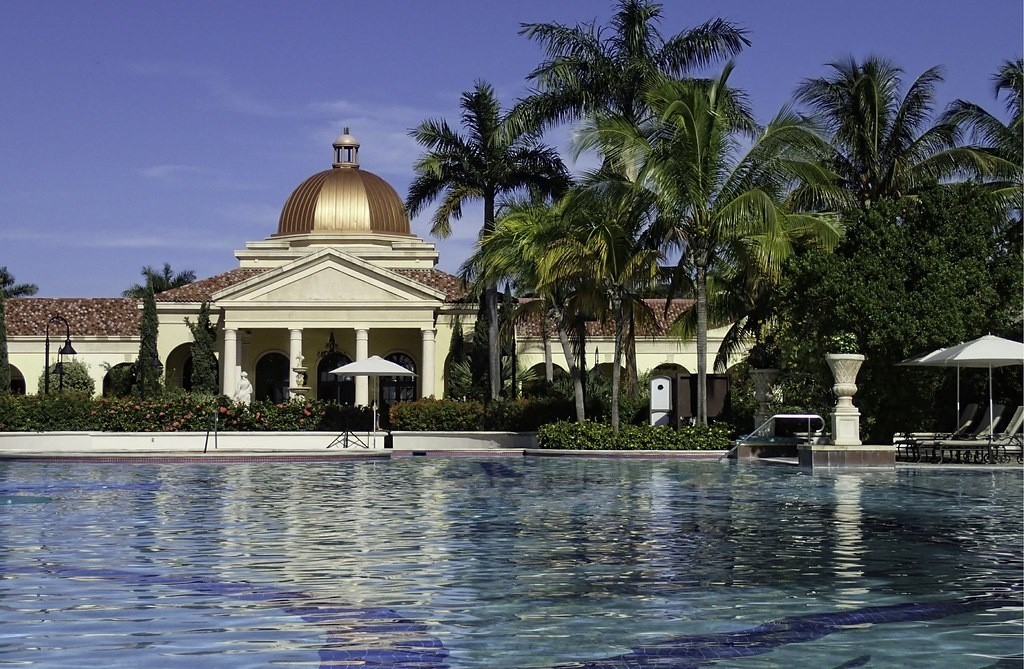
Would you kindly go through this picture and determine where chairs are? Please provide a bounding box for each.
[934,405,1024,464]
[892,404,978,461]
[915,405,1005,462]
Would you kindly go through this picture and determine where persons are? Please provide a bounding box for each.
[232,371,255,412]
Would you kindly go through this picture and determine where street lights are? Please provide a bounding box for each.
[44,312,77,395]
[51,345,68,396]
